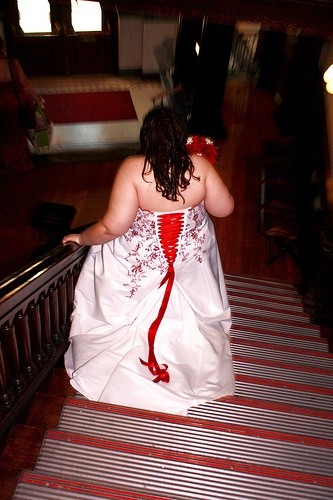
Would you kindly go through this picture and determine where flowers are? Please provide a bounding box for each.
[185,134,221,167]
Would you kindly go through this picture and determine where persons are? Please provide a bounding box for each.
[62,106,236,416]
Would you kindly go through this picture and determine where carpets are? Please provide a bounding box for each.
[40,90,138,124]
